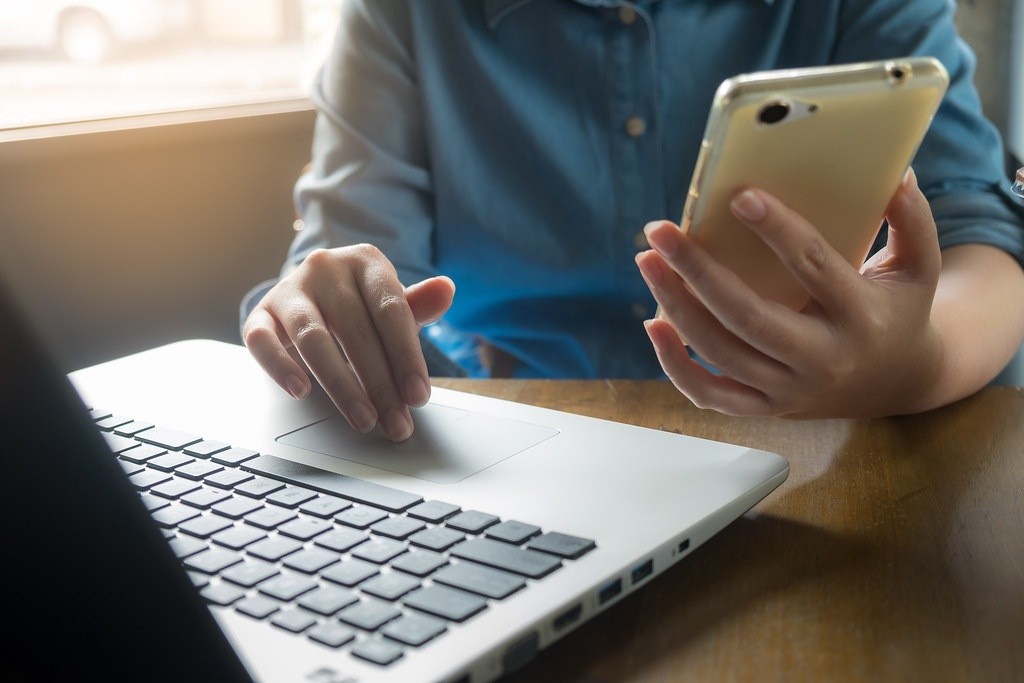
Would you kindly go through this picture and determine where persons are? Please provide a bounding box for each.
[239,0,1024,439]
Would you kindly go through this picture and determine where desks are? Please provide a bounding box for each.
[426,376,1024,683]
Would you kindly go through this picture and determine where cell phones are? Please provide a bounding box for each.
[656,56,949,344]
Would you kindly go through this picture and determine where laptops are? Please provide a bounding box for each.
[1,281,792,683]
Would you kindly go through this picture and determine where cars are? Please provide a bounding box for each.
[0,0,303,63]
